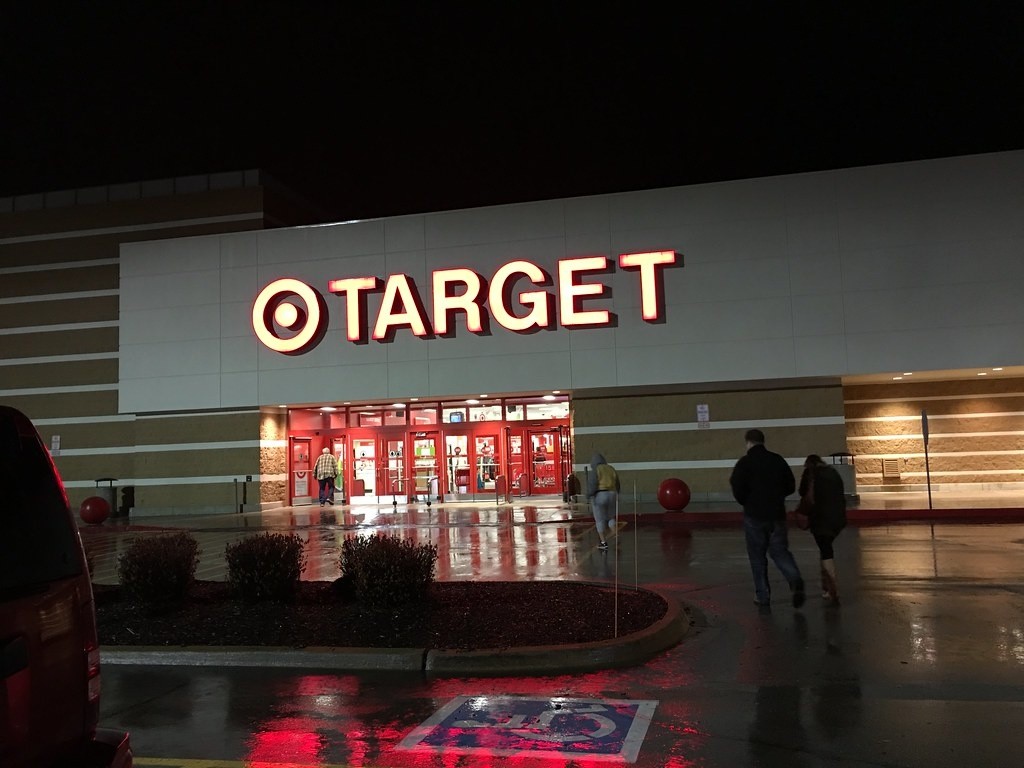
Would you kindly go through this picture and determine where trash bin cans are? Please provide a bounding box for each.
[828,452,860,502]
[94,477,119,520]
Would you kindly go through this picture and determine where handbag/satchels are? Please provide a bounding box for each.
[794,465,816,530]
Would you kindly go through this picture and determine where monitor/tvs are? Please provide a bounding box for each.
[450,412,464,423]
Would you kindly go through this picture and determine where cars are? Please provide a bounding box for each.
[0,404,137,768]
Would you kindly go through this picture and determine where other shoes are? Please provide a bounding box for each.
[320,503,324,507]
[822,587,839,601]
[753,589,770,606]
[596,541,608,549]
[326,498,334,505]
[789,579,804,608]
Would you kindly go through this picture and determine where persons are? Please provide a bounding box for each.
[313,448,339,506]
[586,454,620,549]
[729,428,806,610]
[798,455,846,599]
